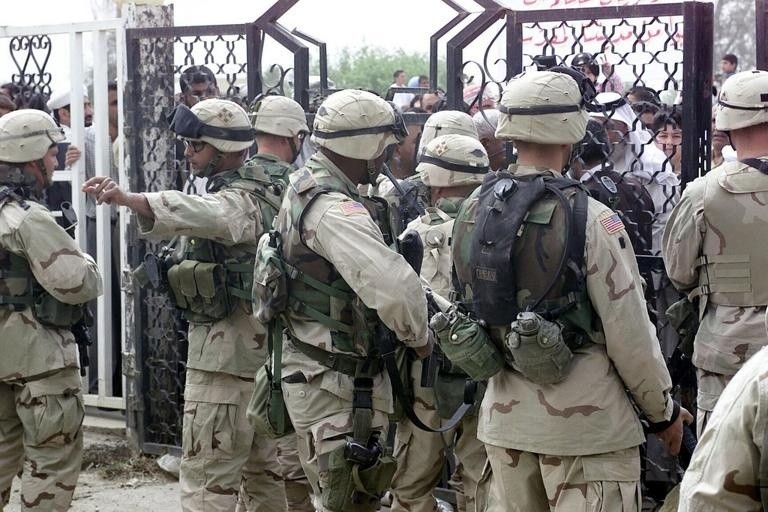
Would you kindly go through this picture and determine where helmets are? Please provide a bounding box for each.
[416,109,500,188]
[248,93,311,137]
[313,89,399,161]
[0,108,67,163]
[715,70,767,130]
[178,100,257,153]
[494,70,591,145]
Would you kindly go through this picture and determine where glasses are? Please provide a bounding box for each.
[183,141,206,151]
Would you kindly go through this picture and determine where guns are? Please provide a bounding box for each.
[61,202,94,376]
[145,253,188,331]
[366,289,451,387]
[383,162,424,232]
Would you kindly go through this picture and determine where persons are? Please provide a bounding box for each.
[1,51,764,511]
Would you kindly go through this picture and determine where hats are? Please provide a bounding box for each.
[48,85,89,111]
